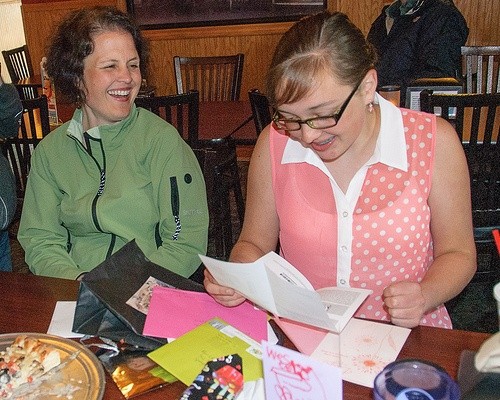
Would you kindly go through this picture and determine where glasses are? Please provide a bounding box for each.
[271,67,374,131]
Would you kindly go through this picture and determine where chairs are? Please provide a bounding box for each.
[184,136,246,288]
[3,96,51,200]
[419,87,500,281]
[171,53,244,99]
[246,86,272,136]
[460,45,500,94]
[1,45,41,109]
[135,89,200,142]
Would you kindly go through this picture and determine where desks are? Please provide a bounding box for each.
[9,96,500,164]
[9,73,41,87]
[0,269,491,400]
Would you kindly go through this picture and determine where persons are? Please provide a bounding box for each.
[204,11,476,329]
[17,6,209,278]
[366,0,469,86]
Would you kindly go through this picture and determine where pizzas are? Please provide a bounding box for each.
[0,335,61,400]
